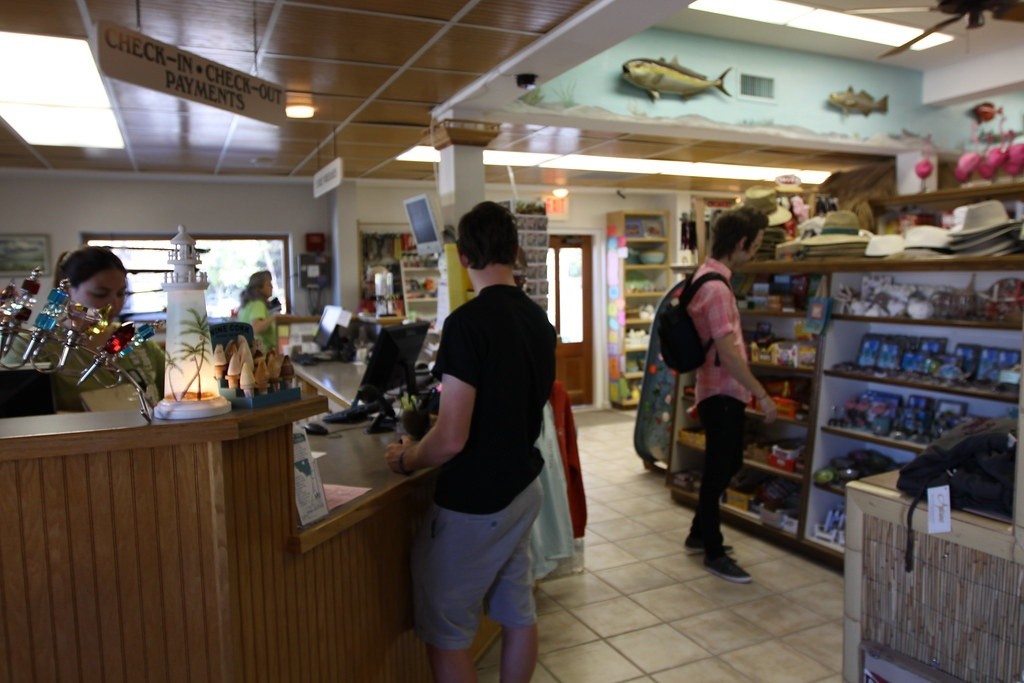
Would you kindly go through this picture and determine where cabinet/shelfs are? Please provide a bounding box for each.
[396,250,439,346]
[606,208,673,406]
[662,252,1024,561]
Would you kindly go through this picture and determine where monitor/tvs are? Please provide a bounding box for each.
[314,305,344,360]
[403,193,443,256]
[357,321,432,405]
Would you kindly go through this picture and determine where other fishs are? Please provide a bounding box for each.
[827,86,889,118]
[622,56,733,103]
[973,103,1003,124]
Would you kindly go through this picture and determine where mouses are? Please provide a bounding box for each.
[304,423,327,436]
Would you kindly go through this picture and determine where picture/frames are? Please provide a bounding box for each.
[0,232,52,277]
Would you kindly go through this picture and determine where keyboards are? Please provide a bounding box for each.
[323,397,395,424]
[295,353,319,363]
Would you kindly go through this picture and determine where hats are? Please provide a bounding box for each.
[864,198,1023,259]
[730,185,875,257]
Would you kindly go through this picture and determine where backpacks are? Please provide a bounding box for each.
[657,268,732,373]
[894,418,1020,572]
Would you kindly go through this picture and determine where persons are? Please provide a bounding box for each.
[681,205,779,585]
[385,200,557,683]
[236,269,280,354]
[0,246,165,414]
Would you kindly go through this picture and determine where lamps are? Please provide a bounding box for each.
[284,91,315,118]
[153,222,231,419]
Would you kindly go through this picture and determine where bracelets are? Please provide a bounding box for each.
[757,392,767,400]
[398,447,415,476]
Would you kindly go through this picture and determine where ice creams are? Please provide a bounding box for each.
[212,334,296,397]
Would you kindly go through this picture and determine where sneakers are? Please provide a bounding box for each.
[701,553,752,583]
[684,534,733,552]
